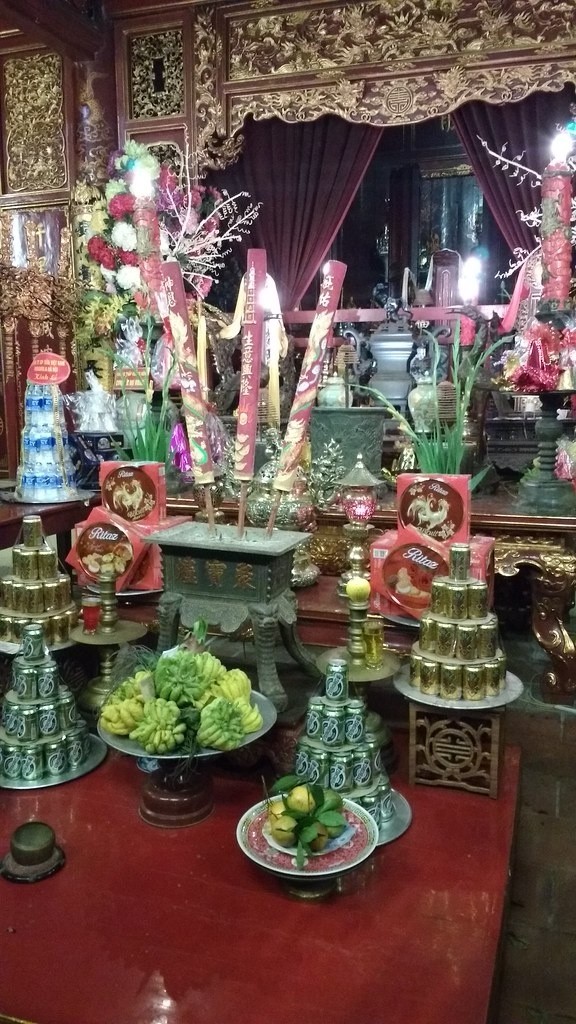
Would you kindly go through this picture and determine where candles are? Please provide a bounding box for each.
[128,162,164,316]
[537,128,574,304]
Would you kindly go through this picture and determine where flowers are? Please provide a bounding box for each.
[65,138,265,375]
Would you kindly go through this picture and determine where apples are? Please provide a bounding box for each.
[346,577,370,603]
[268,785,345,853]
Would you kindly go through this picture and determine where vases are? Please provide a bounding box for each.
[309,407,394,498]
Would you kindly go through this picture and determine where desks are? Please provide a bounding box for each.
[98,491,576,707]
[0,725,526,1024]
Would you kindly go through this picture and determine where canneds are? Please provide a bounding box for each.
[293,659,393,831]
[0,515,79,648]
[410,543,506,702]
[0,624,91,782]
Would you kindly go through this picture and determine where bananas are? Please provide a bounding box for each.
[97,650,263,755]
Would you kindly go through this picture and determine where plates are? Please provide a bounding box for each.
[97,687,278,759]
[235,795,379,876]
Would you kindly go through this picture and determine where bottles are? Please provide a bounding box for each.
[19,382,77,498]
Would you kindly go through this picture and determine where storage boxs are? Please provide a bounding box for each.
[72,514,193,589]
[373,530,496,615]
[394,473,472,543]
[406,704,509,798]
[63,506,150,593]
